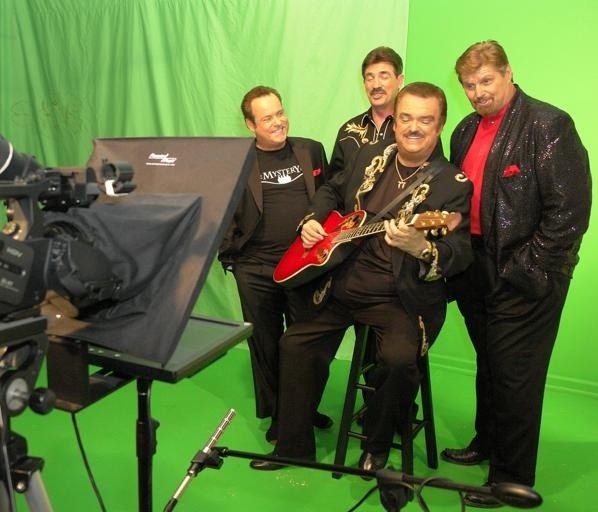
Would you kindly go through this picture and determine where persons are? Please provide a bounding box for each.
[440,40,592,508]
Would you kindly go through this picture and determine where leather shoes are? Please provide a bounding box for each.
[358,450,390,481]
[464,483,502,508]
[249,412,333,470]
[440,443,491,465]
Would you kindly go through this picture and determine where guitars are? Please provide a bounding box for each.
[273,210,462,290]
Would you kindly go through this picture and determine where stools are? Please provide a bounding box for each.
[332,325,437,483]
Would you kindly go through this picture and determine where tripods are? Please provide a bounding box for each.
[0,427,53,512]
